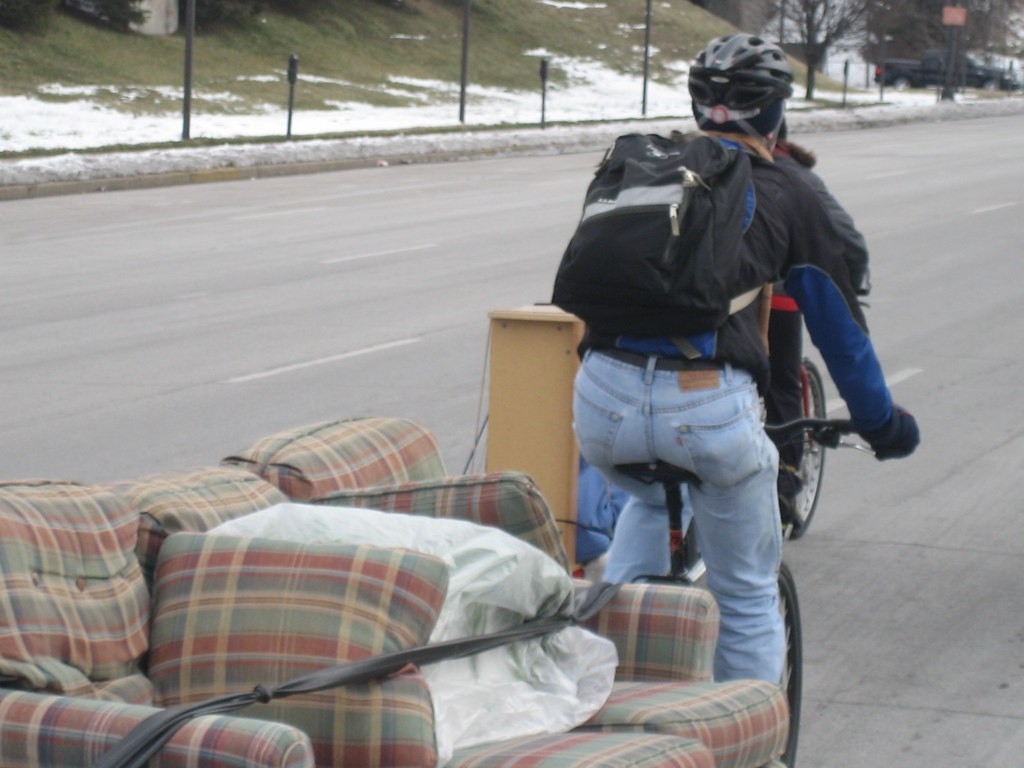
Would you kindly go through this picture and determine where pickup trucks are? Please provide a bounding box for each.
[875,48,1024,91]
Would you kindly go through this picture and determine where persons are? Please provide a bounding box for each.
[574,34,920,683]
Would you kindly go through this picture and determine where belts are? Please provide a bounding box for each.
[592,346,742,371]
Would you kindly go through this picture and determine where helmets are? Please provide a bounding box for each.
[687,32,795,111]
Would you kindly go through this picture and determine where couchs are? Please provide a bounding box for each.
[0,414,794,768]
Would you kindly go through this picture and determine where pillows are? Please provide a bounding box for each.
[314,472,572,578]
[150,530,449,768]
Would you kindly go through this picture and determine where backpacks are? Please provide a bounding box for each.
[550,133,769,338]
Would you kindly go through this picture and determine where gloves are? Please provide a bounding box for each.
[849,402,921,461]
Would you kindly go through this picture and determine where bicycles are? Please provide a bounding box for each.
[613,417,879,767]
[684,286,869,572]
